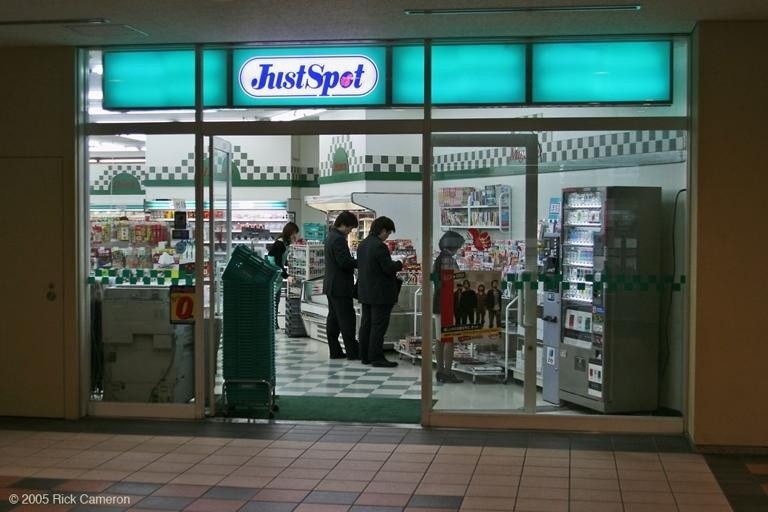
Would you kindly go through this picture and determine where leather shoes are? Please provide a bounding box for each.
[330,353,362,360]
[436,372,463,383]
[362,357,398,367]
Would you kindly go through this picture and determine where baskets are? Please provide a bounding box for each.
[222,245,283,406]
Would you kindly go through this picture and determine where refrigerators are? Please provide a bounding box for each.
[299,190,423,350]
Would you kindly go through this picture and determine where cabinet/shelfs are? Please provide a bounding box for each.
[438,184,513,240]
[89,208,376,282]
[383,284,436,344]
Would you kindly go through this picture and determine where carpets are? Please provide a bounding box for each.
[211,396,437,422]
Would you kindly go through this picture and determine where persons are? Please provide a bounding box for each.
[267,219,300,329]
[353,215,409,367]
[453,283,463,327]
[475,284,488,328]
[487,279,501,329]
[432,230,473,385]
[461,280,476,324]
[319,211,367,360]
